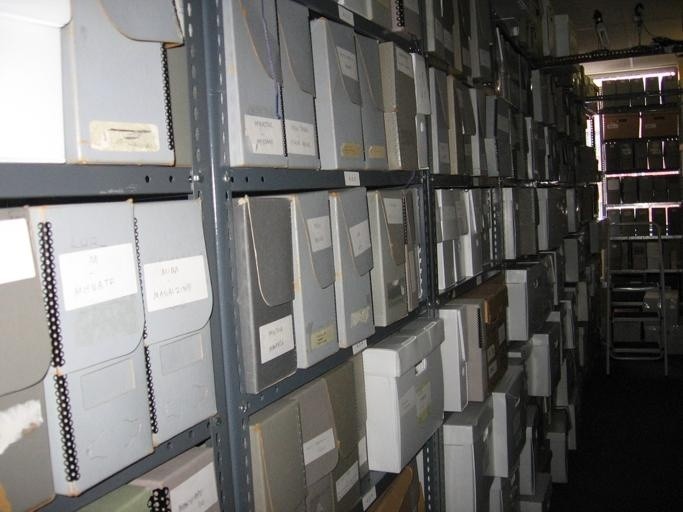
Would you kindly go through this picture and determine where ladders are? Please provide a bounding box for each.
[606,222,670,376]
